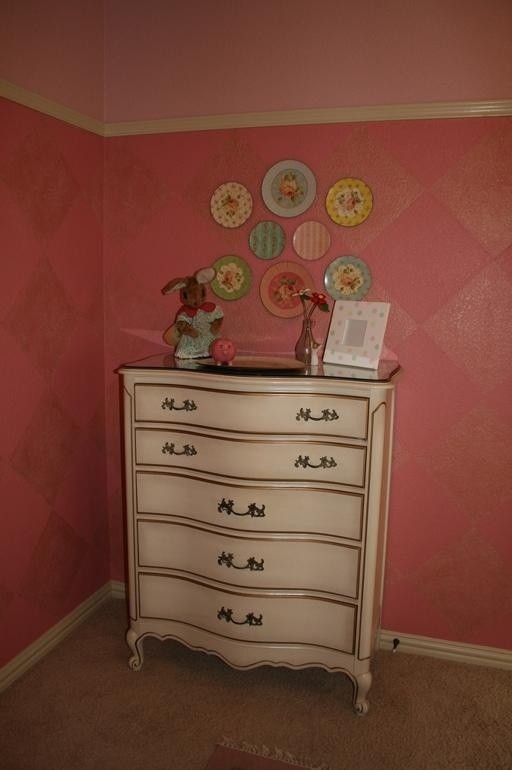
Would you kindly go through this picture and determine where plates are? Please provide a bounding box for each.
[259,261,315,320]
[324,254,371,302]
[208,255,252,302]
[209,181,254,229]
[292,220,331,261]
[261,160,318,219]
[249,221,287,261]
[325,176,374,228]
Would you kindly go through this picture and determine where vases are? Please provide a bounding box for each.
[294,314,317,363]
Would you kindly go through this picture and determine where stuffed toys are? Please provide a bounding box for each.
[157,266,223,359]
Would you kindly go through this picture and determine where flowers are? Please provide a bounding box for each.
[291,285,330,320]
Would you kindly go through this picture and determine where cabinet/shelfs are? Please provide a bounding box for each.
[113,353,400,718]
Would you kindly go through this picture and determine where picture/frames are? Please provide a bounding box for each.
[322,299,390,370]
